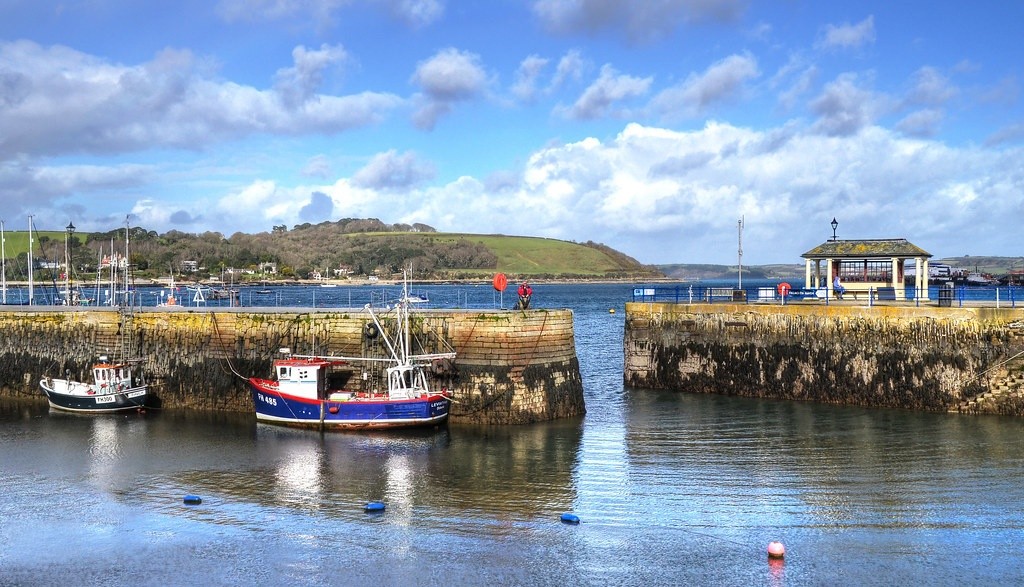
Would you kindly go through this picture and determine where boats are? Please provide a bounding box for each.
[186,279,210,291]
[248,269,457,431]
[38,291,152,414]
[399,262,429,303]
[207,265,240,298]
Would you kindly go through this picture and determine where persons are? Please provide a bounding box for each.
[833,276,844,299]
[950,270,959,281]
[963,270,967,279]
[517,281,533,308]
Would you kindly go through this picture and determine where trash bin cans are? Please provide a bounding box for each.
[939,285,951,307]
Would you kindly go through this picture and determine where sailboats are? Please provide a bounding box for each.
[257,266,273,293]
[320,266,337,287]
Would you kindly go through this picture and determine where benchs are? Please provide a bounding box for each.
[833,282,886,299]
[703,287,733,302]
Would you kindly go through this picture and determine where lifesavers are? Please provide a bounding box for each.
[494,272,507,291]
[365,322,378,337]
[117,382,129,391]
[98,384,112,394]
[778,282,791,296]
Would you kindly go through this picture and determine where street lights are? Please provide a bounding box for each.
[831,217,839,240]
[66,221,76,306]
